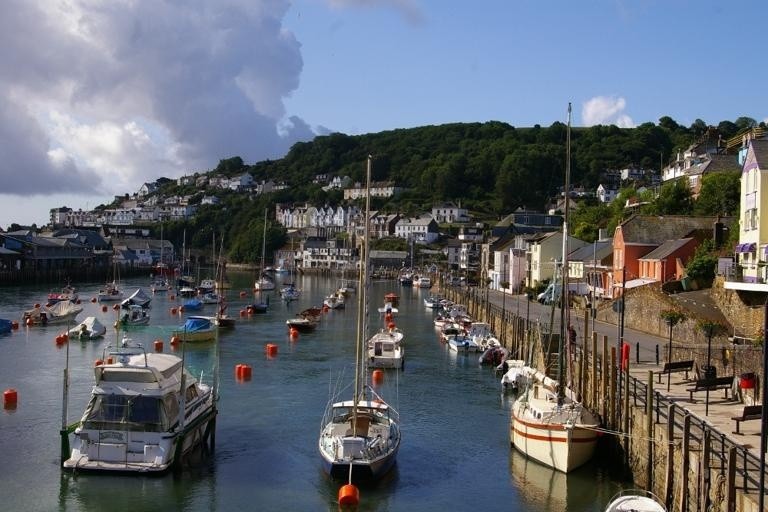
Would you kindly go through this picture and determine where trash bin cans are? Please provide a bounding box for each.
[704,365,716,391]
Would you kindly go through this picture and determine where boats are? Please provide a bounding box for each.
[381,292,401,308]
[422,293,524,393]
[605,486,670,511]
[287,278,356,332]
[395,271,435,289]
[54,302,226,473]
[366,301,408,371]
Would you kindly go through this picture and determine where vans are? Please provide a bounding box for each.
[535,281,592,308]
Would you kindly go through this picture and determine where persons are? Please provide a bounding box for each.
[568,324,577,353]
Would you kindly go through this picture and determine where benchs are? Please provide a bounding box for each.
[653,360,695,385]
[686,376,738,404]
[730,404,764,436]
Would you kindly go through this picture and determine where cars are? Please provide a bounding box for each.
[433,267,479,289]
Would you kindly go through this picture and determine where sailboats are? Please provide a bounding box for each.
[315,150,402,481]
[506,443,599,511]
[512,102,607,472]
